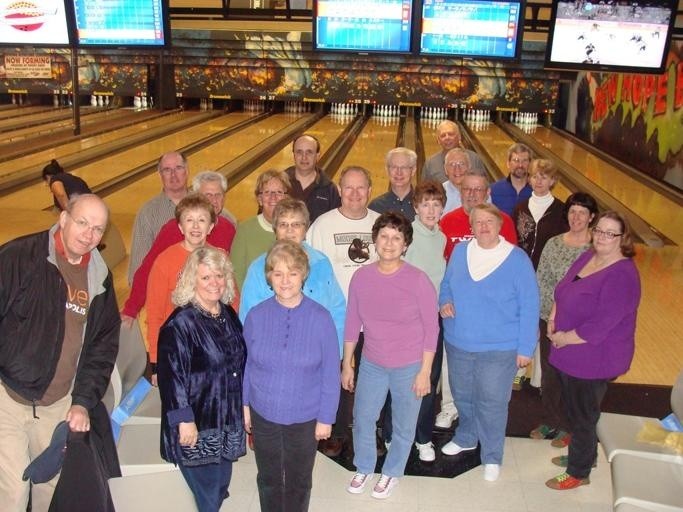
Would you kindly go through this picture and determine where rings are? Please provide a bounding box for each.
[324,437,327,439]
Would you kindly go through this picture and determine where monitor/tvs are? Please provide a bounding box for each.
[544,0,680,75]
[415,0,526,62]
[312,0,416,55]
[67,0,172,50]
[0,0,75,49]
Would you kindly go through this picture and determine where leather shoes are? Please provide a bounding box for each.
[376,438,386,457]
[323,437,342,457]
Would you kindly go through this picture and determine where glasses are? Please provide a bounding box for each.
[67,211,108,233]
[448,161,465,167]
[259,189,288,195]
[592,226,622,239]
[159,167,183,175]
[513,156,529,163]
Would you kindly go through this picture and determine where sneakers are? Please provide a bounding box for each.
[434,403,459,428]
[348,473,373,494]
[546,472,590,490]
[552,455,569,467]
[551,431,574,447]
[415,441,435,462]
[371,475,397,498]
[530,425,556,439]
[485,463,499,481]
[441,441,477,455]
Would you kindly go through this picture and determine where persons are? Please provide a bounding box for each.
[128,151,191,287]
[156,247,246,512]
[232,119,641,497]
[0,193,121,512]
[240,243,341,512]
[119,171,237,328]
[146,194,240,387]
[42,159,107,252]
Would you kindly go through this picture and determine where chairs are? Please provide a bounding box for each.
[595,375,683,512]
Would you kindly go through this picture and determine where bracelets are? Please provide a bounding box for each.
[547,318,555,322]
